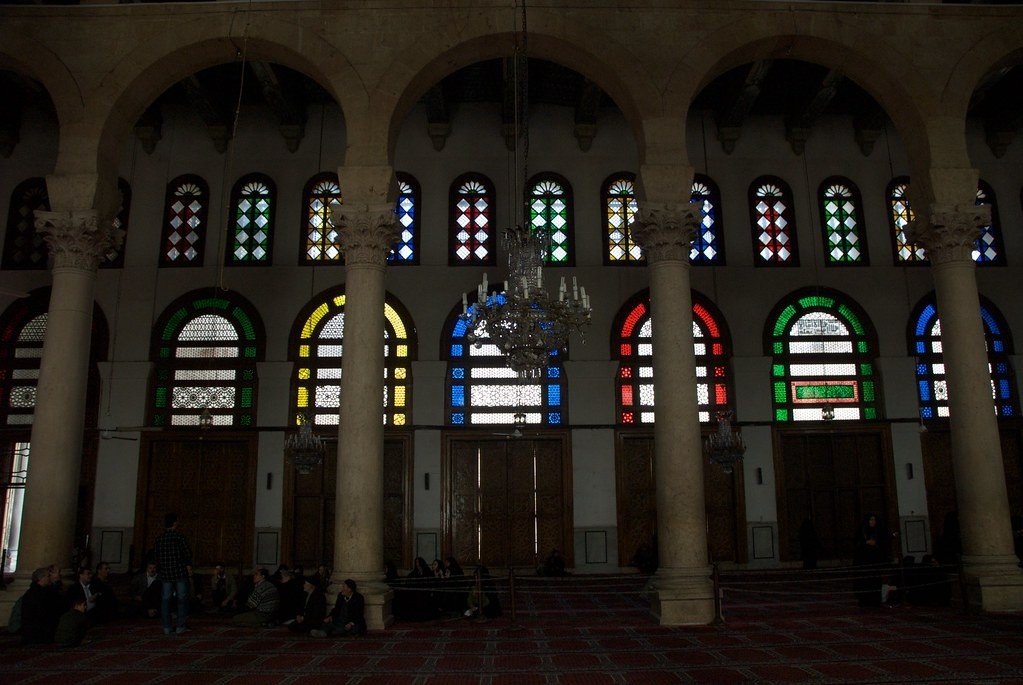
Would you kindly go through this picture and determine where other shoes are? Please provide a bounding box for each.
[164,626,173,634]
[311,628,327,638]
[176,625,187,634]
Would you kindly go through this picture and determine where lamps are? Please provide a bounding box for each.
[704,407,748,483]
[282,411,328,477]
[918,406,927,433]
[98,263,122,439]
[199,407,213,434]
[514,405,526,433]
[822,382,834,423]
[457,0,593,378]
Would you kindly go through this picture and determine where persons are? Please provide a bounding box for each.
[233,564,304,625]
[311,580,367,638]
[408,557,463,578]
[315,565,330,580]
[71,567,100,626]
[141,563,162,617]
[212,563,237,614]
[155,514,193,634]
[186,564,203,614]
[856,514,899,562]
[934,511,962,562]
[284,576,327,632]
[90,562,126,620]
[20,565,71,642]
